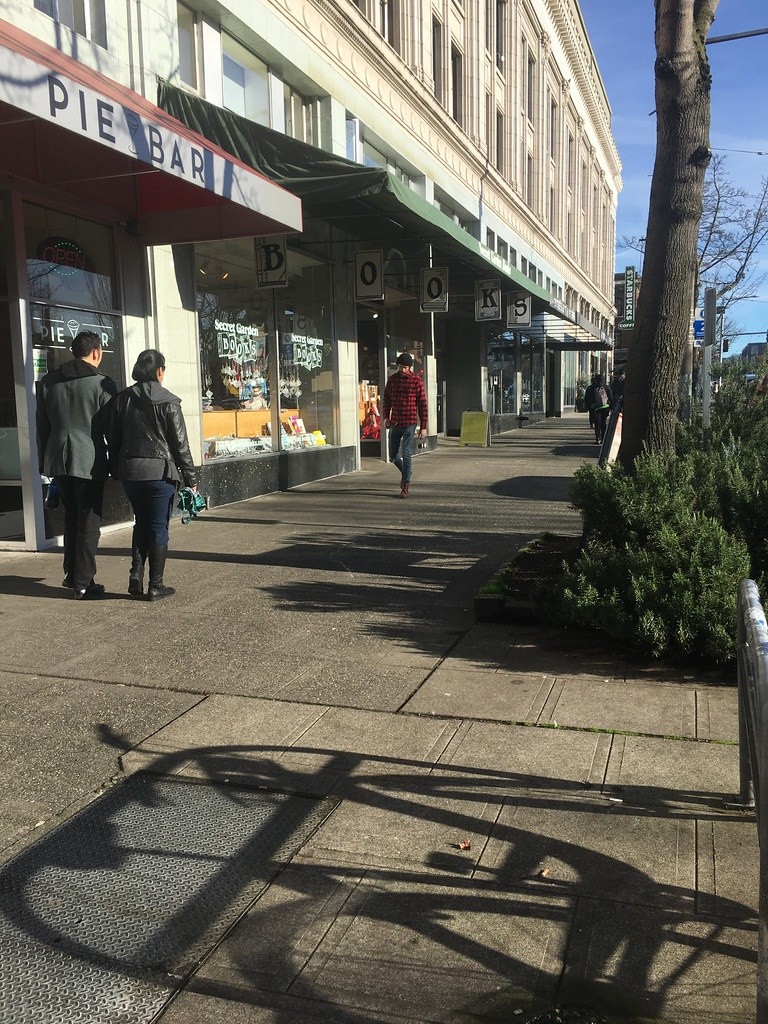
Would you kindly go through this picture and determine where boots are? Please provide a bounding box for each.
[127,525,148,596]
[149,543,176,602]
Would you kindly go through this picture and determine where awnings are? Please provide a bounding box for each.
[0,18,302,248]
[156,74,550,328]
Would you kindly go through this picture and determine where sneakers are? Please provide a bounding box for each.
[398,481,410,499]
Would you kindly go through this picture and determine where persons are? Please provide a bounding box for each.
[383,354,428,498]
[584,370,625,446]
[35,331,116,600]
[109,349,198,601]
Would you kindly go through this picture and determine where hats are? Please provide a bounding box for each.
[397,353,413,366]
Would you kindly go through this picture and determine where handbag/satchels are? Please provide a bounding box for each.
[178,486,206,526]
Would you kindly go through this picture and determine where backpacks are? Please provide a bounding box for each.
[592,382,611,412]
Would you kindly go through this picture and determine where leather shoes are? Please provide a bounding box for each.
[63,571,88,590]
[73,580,105,601]
